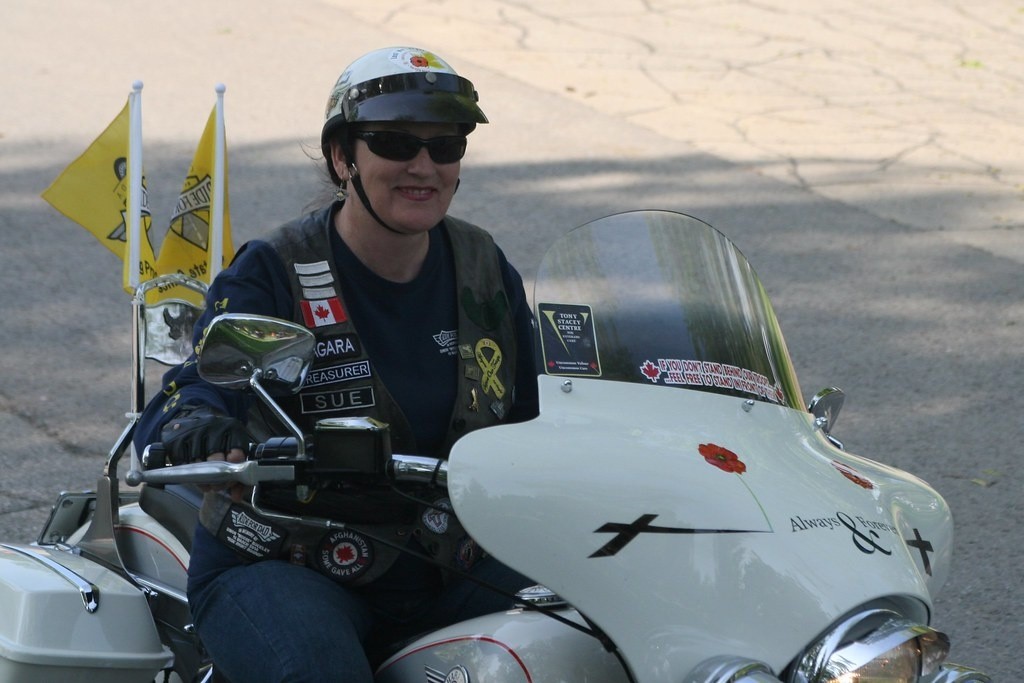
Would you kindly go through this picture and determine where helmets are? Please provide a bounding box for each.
[318,44,490,166]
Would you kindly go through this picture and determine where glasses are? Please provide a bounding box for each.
[351,128,468,165]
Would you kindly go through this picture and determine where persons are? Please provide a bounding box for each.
[135,46,536,683]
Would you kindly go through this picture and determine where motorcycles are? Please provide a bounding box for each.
[0,206,990,683]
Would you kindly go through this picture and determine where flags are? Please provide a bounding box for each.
[44,89,233,316]
